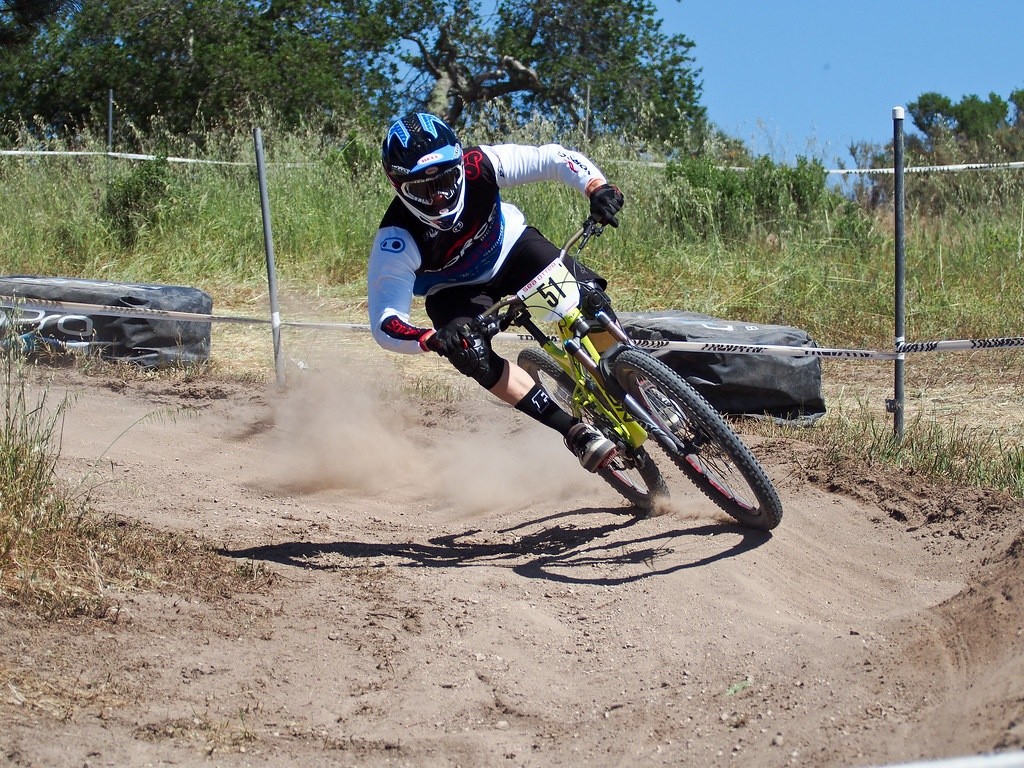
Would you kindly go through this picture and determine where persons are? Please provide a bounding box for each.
[368,114,680,473]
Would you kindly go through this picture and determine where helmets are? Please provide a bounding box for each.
[382,114,465,231]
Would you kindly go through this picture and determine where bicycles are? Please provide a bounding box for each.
[457,218,783,532]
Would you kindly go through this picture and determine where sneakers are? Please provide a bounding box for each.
[563,423,617,472]
[641,382,695,442]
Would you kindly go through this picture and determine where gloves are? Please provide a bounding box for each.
[590,185,623,228]
[435,317,481,358]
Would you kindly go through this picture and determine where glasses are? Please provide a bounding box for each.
[401,164,464,206]
